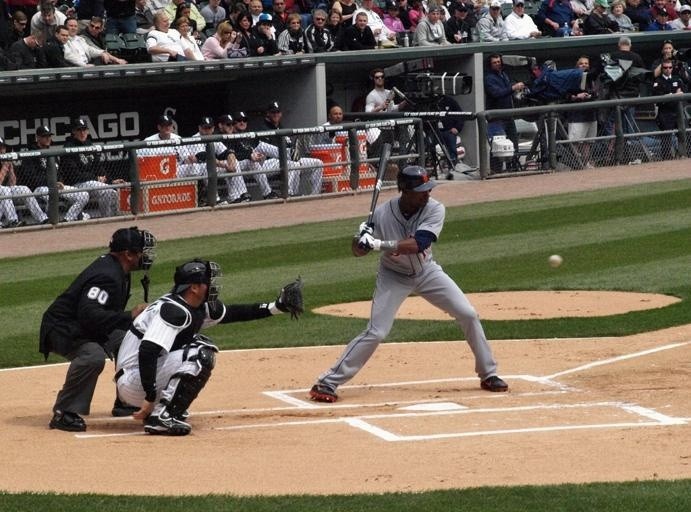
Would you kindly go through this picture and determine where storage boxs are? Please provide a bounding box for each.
[317,170,378,195]
[118,179,201,216]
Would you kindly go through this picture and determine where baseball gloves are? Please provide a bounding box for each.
[276,274,305,321]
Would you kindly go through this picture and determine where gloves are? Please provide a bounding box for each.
[354,220,384,256]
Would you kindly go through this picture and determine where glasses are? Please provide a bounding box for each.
[90,23,102,30]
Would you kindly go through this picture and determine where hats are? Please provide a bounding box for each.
[154,100,306,127]
[655,4,690,13]
[597,0,611,9]
[401,1,539,18]
[260,14,272,21]
[387,2,399,11]
[71,119,89,132]
[36,124,54,137]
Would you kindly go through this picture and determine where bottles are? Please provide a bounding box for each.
[404,34,410,47]
[378,39,383,50]
[563,22,570,39]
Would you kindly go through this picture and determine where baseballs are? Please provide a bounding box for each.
[549,254,561,266]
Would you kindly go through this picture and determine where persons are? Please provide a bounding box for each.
[39,226,158,432]
[113,260,304,436]
[310,165,508,403]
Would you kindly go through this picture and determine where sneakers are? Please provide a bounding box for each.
[109,399,192,437]
[308,383,339,404]
[199,190,279,205]
[48,407,87,436]
[479,373,511,394]
[0,214,92,227]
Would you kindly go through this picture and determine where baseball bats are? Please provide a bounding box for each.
[358,143,392,251]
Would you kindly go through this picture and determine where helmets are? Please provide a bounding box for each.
[171,256,213,300]
[397,163,437,195]
[108,227,156,273]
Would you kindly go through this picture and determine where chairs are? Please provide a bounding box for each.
[124,33,150,63]
[298,13,310,31]
[4,164,99,213]
[104,33,137,65]
[205,27,218,38]
[477,0,542,25]
[194,32,208,49]
[513,118,543,171]
[124,142,285,199]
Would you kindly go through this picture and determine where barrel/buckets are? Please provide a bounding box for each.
[134,146,177,180]
[309,129,378,192]
